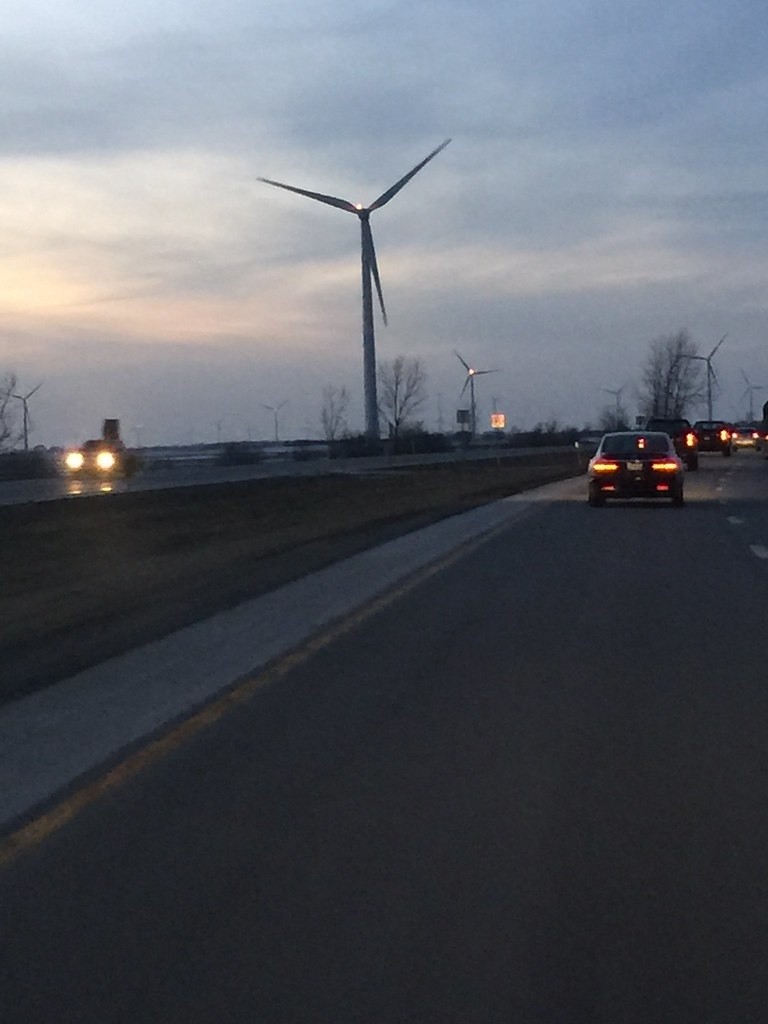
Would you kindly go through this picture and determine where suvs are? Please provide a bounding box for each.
[693,420,735,457]
[644,417,703,471]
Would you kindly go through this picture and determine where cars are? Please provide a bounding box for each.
[66,440,129,482]
[731,426,762,452]
[584,431,689,508]
